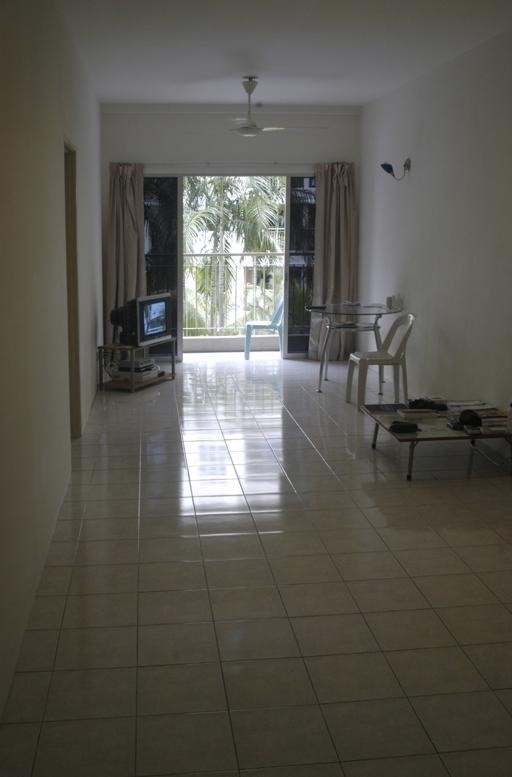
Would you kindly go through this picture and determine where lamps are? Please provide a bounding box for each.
[380,158,410,181]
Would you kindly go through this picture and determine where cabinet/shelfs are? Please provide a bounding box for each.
[143,177,183,361]
[282,176,316,360]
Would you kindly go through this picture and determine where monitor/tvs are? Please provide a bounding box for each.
[109,291,173,348]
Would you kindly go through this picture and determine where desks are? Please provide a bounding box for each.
[360,401,512,481]
[98,337,176,393]
[304,303,403,395]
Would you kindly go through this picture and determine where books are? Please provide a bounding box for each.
[397,408,441,419]
[445,400,508,435]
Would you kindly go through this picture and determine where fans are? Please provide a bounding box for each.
[185,76,328,137]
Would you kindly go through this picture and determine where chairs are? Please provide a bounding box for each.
[245,297,284,360]
[346,313,415,412]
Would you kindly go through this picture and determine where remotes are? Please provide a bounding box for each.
[307,304,330,310]
